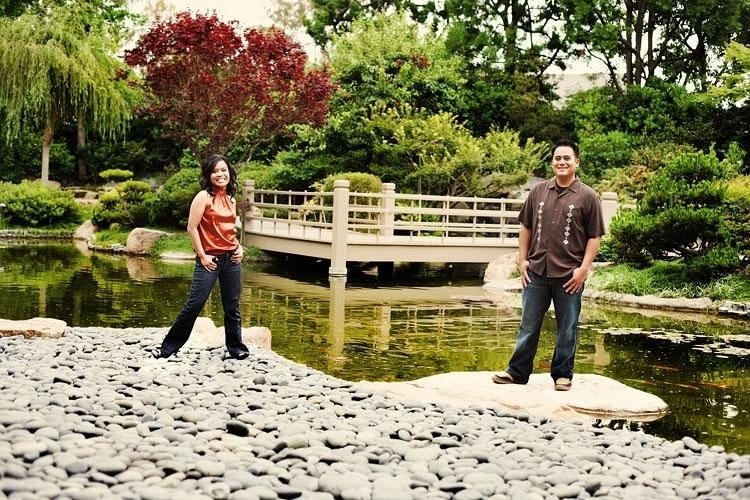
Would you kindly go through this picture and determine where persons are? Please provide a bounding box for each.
[151,154,250,360]
[491,144,605,391]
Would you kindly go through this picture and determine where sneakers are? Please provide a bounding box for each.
[227,345,249,361]
[554,377,572,391]
[492,371,529,385]
[151,349,170,358]
